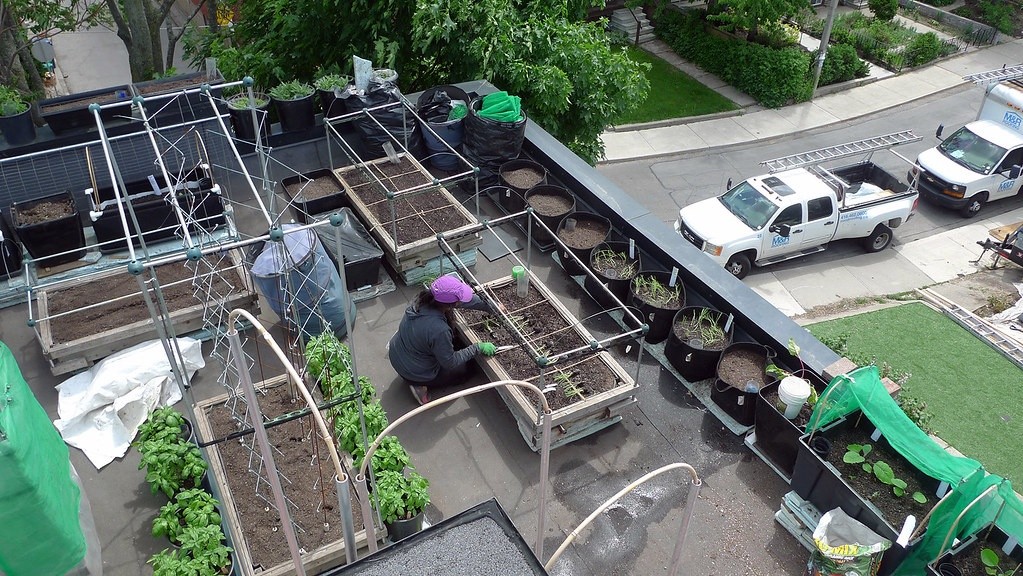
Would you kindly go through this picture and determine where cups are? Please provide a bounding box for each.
[517,273,528,298]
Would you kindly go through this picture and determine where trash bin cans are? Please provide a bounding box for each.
[342,66,428,168]
[415,84,471,172]
[460,95,528,195]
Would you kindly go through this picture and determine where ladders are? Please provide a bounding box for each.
[758,129,924,174]
[962,65,1023,85]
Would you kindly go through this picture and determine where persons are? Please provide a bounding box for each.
[387,272,495,406]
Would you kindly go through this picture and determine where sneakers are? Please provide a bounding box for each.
[409,383,430,405]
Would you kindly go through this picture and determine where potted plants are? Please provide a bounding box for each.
[304,332,429,542]
[0,85,35,145]
[131,405,236,576]
[316,74,353,117]
[589,241,642,306]
[925,518,1023,576]
[626,269,686,343]
[134,68,226,114]
[227,92,271,142]
[790,406,956,576]
[269,79,315,132]
[666,306,734,381]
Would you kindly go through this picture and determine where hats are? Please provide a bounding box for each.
[431,274,473,304]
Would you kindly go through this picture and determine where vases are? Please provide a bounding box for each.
[280,167,346,223]
[88,165,226,254]
[37,84,135,134]
[7,190,87,267]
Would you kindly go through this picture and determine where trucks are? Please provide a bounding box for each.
[907,80,1023,219]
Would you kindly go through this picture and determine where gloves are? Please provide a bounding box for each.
[477,342,496,357]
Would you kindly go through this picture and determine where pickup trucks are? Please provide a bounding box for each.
[674,160,919,281]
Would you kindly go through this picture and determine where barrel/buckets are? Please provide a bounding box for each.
[244,229,356,348]
[775,377,810,419]
